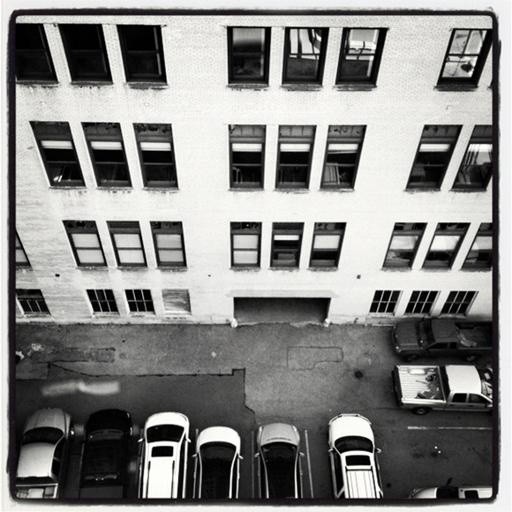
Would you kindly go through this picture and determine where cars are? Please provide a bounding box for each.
[137,412,191,500]
[410,486,494,500]
[191,427,244,499]
[78,408,133,499]
[327,414,384,500]
[254,424,306,500]
[390,319,493,362]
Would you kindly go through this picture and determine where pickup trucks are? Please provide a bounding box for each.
[14,405,75,500]
[391,365,494,417]
